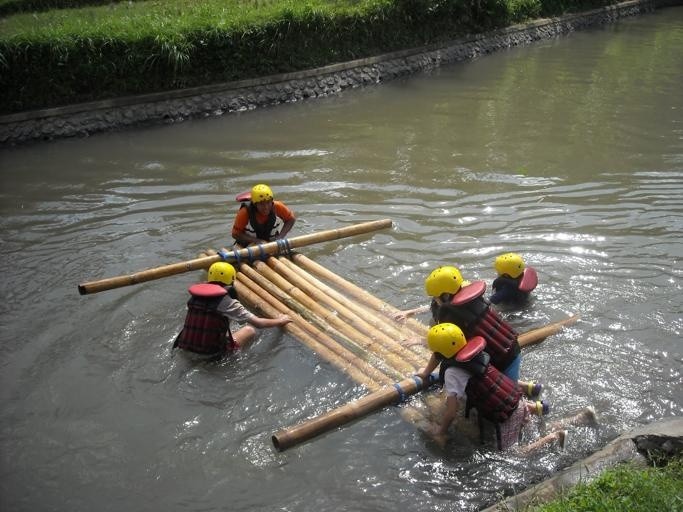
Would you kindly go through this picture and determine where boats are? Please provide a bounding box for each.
[76,215,594,469]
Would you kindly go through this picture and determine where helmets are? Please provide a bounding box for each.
[427,323,467,359]
[208,262,236,285]
[425,266,464,297]
[494,253,525,279]
[251,184,274,204]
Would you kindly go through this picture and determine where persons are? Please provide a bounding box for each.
[392,265,549,416]
[171,261,294,363]
[487,253,538,306]
[427,323,600,455]
[231,184,296,248]
